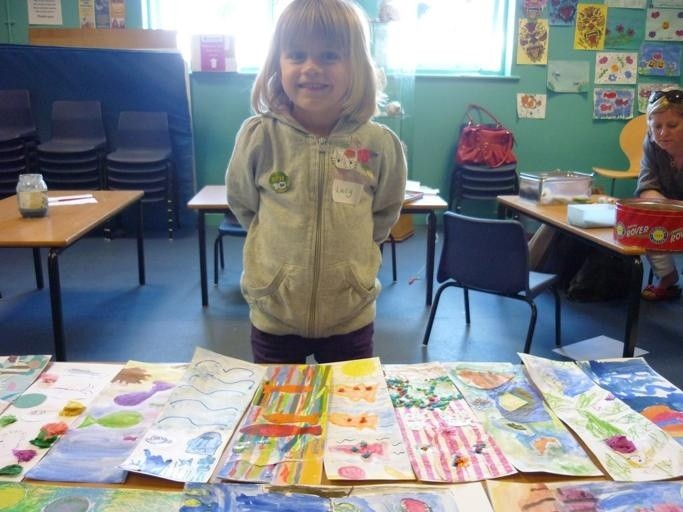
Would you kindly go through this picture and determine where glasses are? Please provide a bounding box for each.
[649,90,682,102]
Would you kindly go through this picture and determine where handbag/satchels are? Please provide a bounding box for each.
[456,125,516,167]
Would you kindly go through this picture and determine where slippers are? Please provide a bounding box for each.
[642,285,679,300]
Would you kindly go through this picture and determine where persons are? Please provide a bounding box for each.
[633,87,683,306]
[218,0,414,370]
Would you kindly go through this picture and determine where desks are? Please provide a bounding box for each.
[0,190,147,362]
[0,352,683,512]
[495,193,683,358]
[186,185,449,305]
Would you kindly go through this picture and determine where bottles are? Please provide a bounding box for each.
[14,172,49,218]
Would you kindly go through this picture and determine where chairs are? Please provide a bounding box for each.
[376,213,415,279]
[448,122,521,210]
[591,113,652,198]
[0,87,181,241]
[214,211,248,286]
[422,211,561,363]
